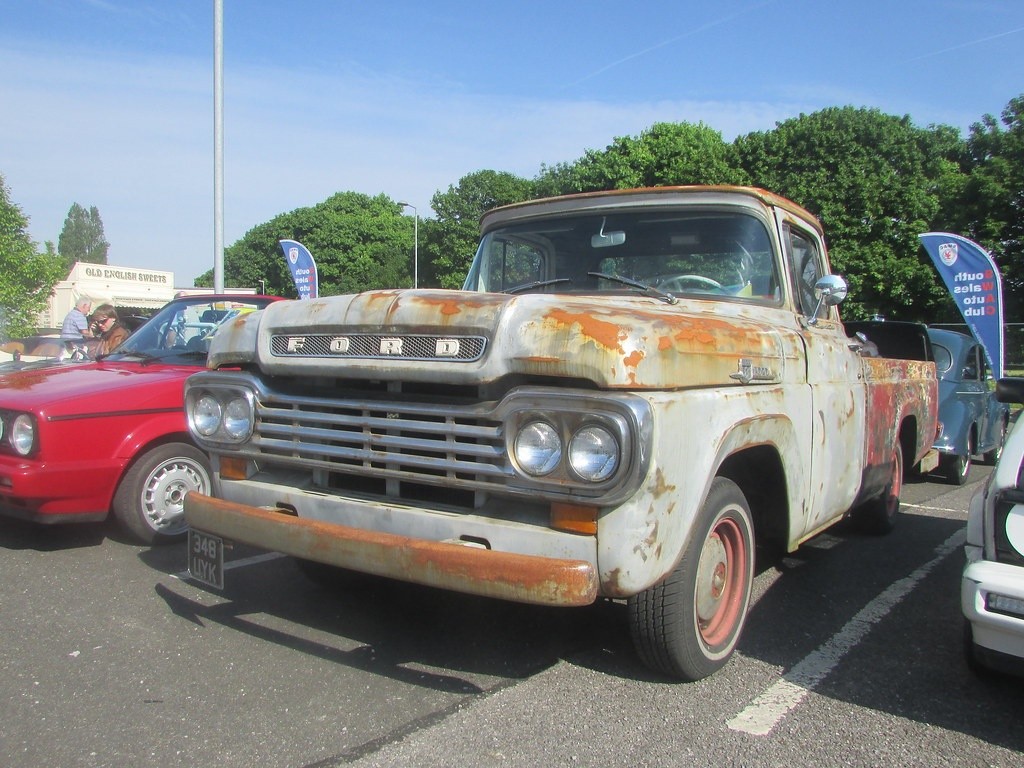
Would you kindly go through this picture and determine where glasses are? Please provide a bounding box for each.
[96,316,109,325]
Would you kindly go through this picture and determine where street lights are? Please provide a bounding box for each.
[258,279,264,295]
[396,200,418,290]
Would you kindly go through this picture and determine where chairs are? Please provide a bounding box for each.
[750,276,776,296]
[187,336,209,353]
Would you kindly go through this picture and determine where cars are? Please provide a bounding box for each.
[1,312,152,372]
[0,293,286,547]
[960,377,1023,690]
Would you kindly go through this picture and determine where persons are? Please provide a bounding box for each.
[90,304,130,359]
[164,291,200,350]
[62,295,96,338]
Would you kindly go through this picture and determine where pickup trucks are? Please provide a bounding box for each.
[184,182,945,682]
[926,327,1011,485]
